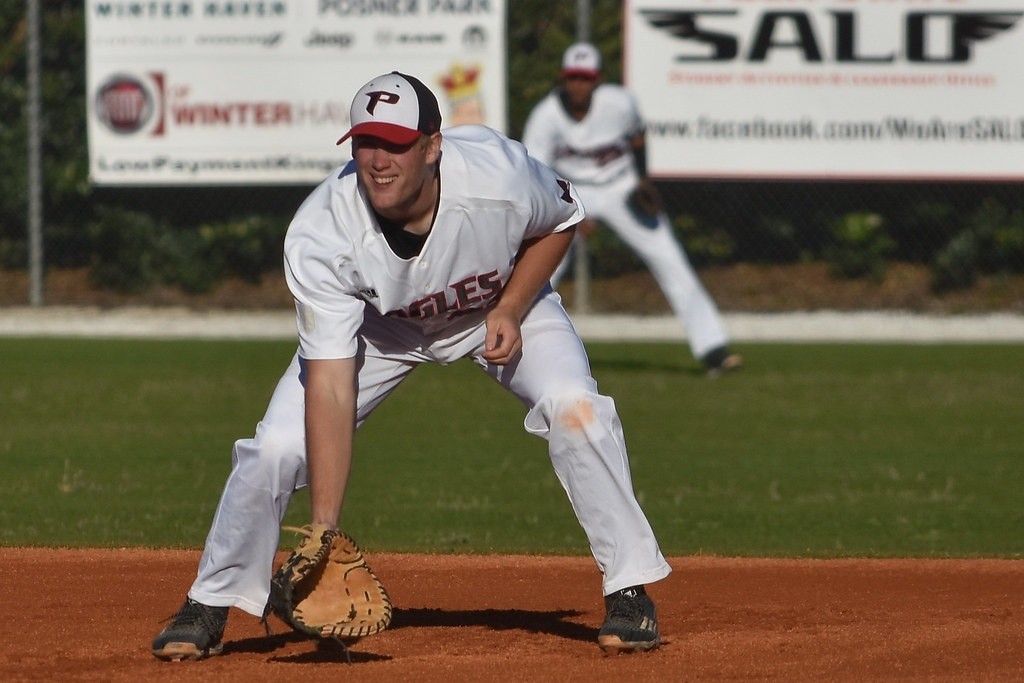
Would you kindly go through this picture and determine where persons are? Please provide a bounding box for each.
[520,43,742,375]
[149,70,673,663]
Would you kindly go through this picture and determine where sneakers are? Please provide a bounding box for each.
[599,587,659,648]
[152,597,229,662]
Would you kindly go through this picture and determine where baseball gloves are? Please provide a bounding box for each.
[258,522,393,665]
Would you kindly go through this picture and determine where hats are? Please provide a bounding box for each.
[560,42,602,79]
[334,70,442,145]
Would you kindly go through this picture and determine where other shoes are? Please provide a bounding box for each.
[706,349,742,380]
